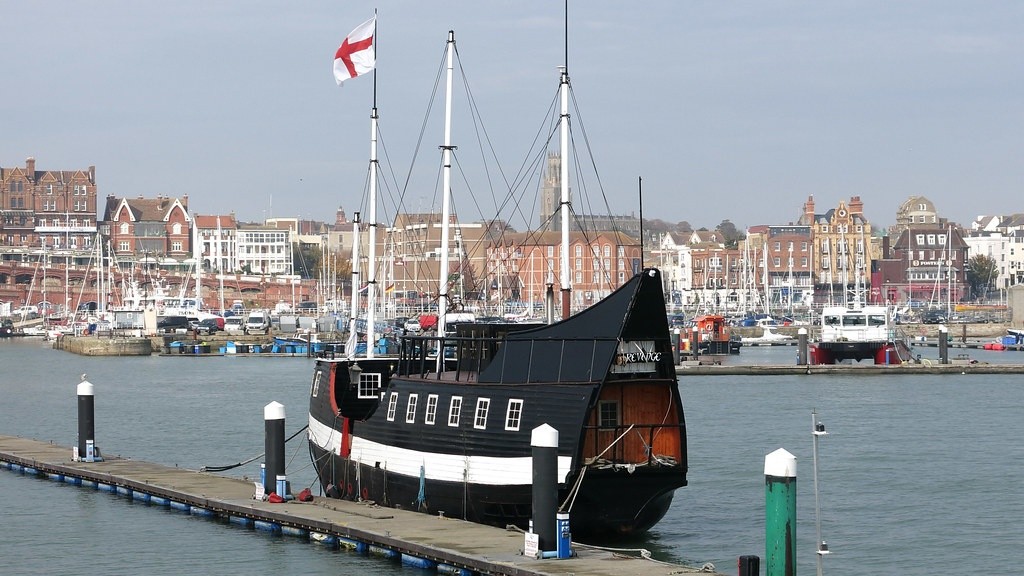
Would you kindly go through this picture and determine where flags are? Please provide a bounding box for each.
[333,17,376,87]
[385,284,395,293]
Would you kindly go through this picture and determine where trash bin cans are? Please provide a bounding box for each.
[271,344,320,353]
[254,345,261,353]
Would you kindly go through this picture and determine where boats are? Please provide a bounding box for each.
[311,265,688,545]
[6,293,1017,366]
